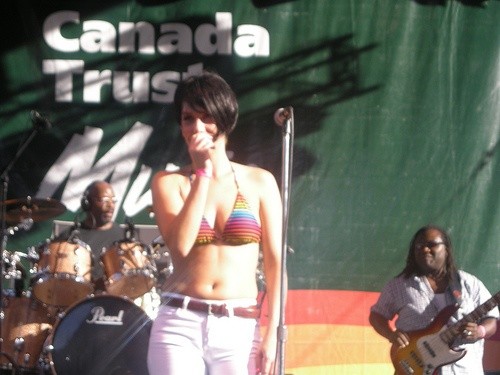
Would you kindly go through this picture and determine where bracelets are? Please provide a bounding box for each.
[479,325,486,338]
[195,169,212,177]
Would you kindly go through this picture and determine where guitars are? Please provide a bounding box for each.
[389,290,500,375]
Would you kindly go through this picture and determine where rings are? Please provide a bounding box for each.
[468,331,472,336]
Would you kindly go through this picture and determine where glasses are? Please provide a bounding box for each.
[98,196,117,203]
[414,241,445,251]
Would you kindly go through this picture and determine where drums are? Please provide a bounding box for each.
[0,289,57,373]
[37,293,155,375]
[94,237,159,301]
[30,236,97,308]
[153,246,175,291]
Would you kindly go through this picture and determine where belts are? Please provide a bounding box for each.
[160,295,261,318]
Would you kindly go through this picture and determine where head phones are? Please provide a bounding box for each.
[80,184,93,213]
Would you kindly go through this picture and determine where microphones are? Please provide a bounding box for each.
[274,106,295,127]
[34,110,52,132]
[125,217,136,234]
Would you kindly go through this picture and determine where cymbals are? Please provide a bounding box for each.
[0,197,68,223]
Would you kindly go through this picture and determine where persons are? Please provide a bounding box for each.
[147,71,288,375]
[369,226,500,375]
[58,180,125,291]
